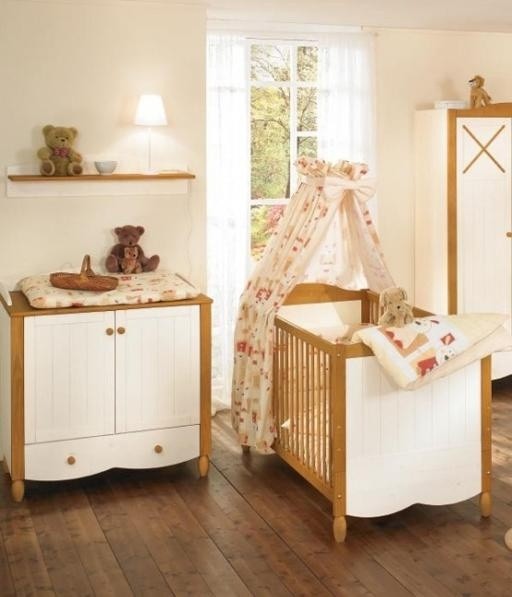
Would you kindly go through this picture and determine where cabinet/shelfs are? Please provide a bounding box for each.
[414,103,511,380]
[0,284,215,504]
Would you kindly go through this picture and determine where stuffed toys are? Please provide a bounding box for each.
[39,123,84,175]
[106,224,160,272]
[376,287,412,324]
[469,75,491,108]
[120,245,142,273]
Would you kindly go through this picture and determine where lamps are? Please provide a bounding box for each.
[132,92,167,171]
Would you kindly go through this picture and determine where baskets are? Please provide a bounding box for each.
[49,254,119,291]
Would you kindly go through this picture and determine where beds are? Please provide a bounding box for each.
[239,278,499,542]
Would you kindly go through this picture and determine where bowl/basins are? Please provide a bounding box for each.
[95,160,119,175]
